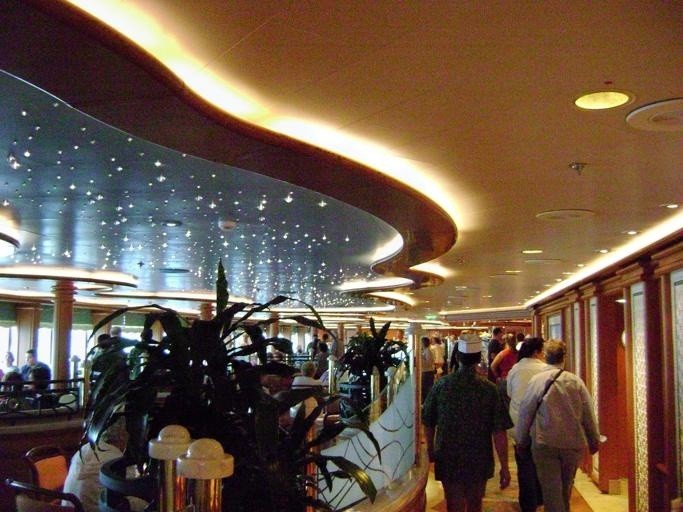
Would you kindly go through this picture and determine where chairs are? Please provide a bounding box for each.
[2,442,85,511]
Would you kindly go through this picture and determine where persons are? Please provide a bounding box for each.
[2,327,602,512]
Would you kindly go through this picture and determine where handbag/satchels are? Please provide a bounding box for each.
[524,434,532,449]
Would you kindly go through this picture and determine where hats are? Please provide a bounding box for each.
[458,334,481,353]
